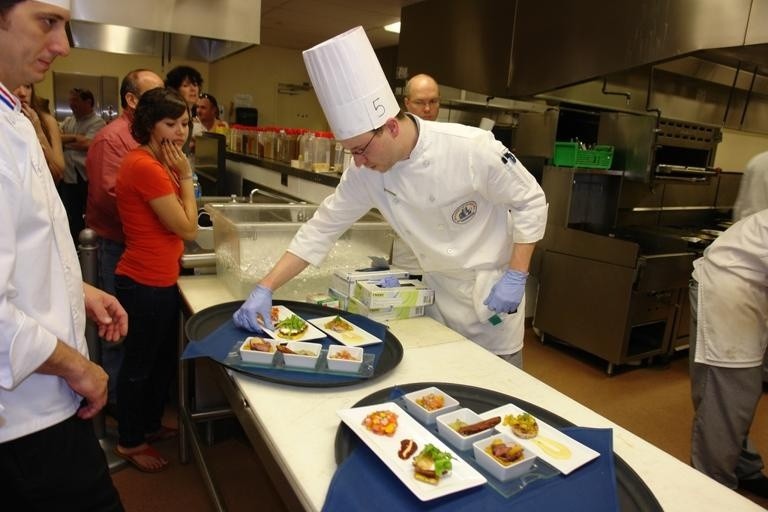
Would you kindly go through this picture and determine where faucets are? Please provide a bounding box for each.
[246,188,260,203]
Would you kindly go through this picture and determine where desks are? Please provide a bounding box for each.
[172,270,767,512]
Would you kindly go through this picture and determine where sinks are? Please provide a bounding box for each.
[577,224,691,259]
[234,197,289,206]
[196,199,231,209]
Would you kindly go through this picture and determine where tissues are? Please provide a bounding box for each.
[333,255,410,297]
[354,275,434,307]
[348,296,424,322]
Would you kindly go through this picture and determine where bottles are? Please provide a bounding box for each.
[192,172,201,207]
[218,122,350,175]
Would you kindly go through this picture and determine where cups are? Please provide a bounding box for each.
[480,118,495,133]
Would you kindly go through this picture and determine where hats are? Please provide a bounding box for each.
[301,24,400,142]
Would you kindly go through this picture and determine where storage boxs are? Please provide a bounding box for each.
[199,193,397,302]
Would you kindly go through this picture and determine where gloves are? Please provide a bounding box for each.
[232,283,275,334]
[483,269,529,314]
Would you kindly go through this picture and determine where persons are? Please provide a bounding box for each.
[689,209,768,497]
[233,109,549,370]
[390,74,440,281]
[12,67,233,250]
[114,87,198,472]
[85,70,165,443]
[0,0,130,512]
[732,151,768,393]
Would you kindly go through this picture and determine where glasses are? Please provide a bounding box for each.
[341,132,377,156]
[198,93,208,99]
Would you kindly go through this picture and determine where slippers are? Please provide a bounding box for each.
[112,443,170,473]
[145,425,178,443]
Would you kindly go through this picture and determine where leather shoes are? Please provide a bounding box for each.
[739,475,768,499]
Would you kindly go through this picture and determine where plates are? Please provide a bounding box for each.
[477,401,601,477]
[256,305,326,345]
[335,401,488,503]
[307,315,382,347]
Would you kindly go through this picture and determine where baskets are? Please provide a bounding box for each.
[552,141,615,170]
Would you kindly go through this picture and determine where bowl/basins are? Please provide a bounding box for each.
[473,432,538,481]
[436,407,495,451]
[403,386,460,425]
[239,336,278,365]
[283,341,322,368]
[327,344,364,372]
